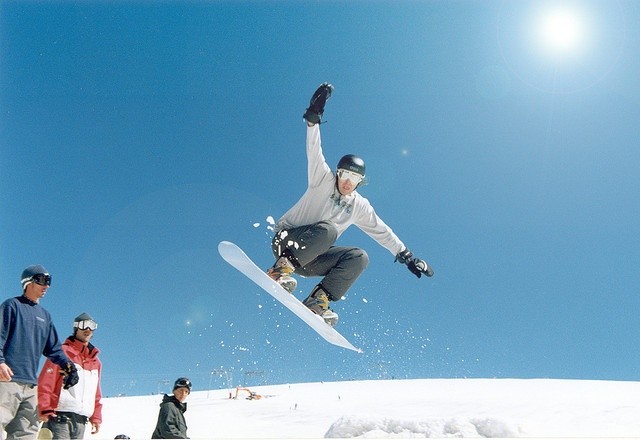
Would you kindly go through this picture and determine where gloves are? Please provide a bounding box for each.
[303,82,334,124]
[60,362,80,389]
[399,252,434,279]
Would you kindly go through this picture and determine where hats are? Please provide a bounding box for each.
[22,265,48,288]
[72,312,95,331]
[174,377,192,390]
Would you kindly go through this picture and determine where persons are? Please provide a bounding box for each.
[149,375,192,438]
[37,313,103,439]
[266,81,434,326]
[0,263,80,440]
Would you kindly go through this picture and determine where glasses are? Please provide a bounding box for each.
[76,321,96,331]
[338,169,363,183]
[29,275,53,286]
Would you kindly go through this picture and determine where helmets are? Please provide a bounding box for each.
[337,154,368,174]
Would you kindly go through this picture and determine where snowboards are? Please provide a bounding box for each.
[217,241,363,353]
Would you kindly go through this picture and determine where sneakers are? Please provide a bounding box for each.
[266,271,296,291]
[303,300,339,324]
